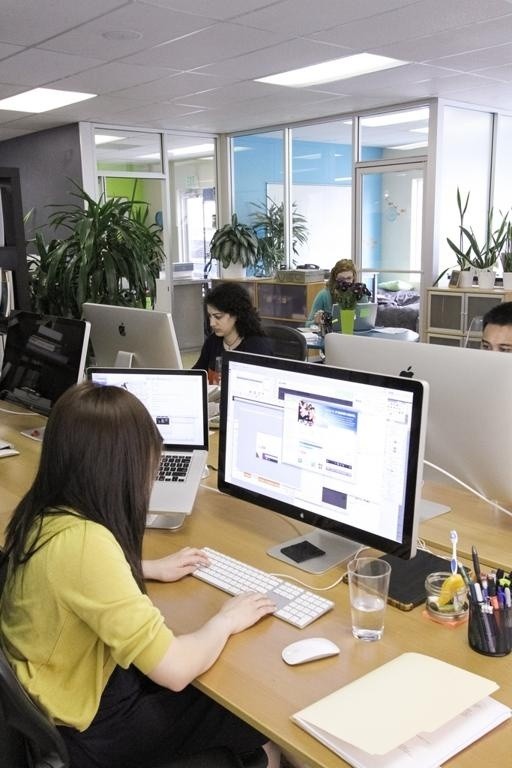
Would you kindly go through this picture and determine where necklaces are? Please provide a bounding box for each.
[223,335,240,350]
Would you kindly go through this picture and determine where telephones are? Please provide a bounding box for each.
[297,263,320,270]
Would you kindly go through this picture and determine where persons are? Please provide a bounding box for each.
[190,281,274,385]
[1,380,279,767]
[304,258,368,328]
[481,302,512,353]
[297,400,315,424]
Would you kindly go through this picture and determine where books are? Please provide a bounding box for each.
[289,650,512,768]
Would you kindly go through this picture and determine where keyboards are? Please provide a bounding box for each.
[188,545,336,630]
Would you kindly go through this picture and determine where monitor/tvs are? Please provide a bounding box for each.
[81,302,183,368]
[217,348,431,575]
[324,332,512,523]
[0,308,91,441]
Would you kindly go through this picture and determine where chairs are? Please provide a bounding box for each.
[0,649,120,768]
[254,325,308,362]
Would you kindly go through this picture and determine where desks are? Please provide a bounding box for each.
[295,324,420,348]
[0,372,512,767]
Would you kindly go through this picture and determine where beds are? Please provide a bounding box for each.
[377,286,419,332]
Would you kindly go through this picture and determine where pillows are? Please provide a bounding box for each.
[376,277,420,305]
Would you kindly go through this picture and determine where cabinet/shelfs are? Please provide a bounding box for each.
[422,287,512,350]
[209,277,327,358]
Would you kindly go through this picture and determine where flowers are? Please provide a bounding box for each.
[334,278,371,310]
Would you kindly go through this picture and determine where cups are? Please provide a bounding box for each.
[347,556,392,642]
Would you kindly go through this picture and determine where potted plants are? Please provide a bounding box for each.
[430,186,512,290]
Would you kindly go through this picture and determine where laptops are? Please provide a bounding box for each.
[86,366,209,516]
[332,303,379,330]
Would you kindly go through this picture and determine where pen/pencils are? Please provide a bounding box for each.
[457,545,512,656]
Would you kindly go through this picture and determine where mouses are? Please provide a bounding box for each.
[281,636,341,665]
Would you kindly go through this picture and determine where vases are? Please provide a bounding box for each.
[341,308,354,335]
